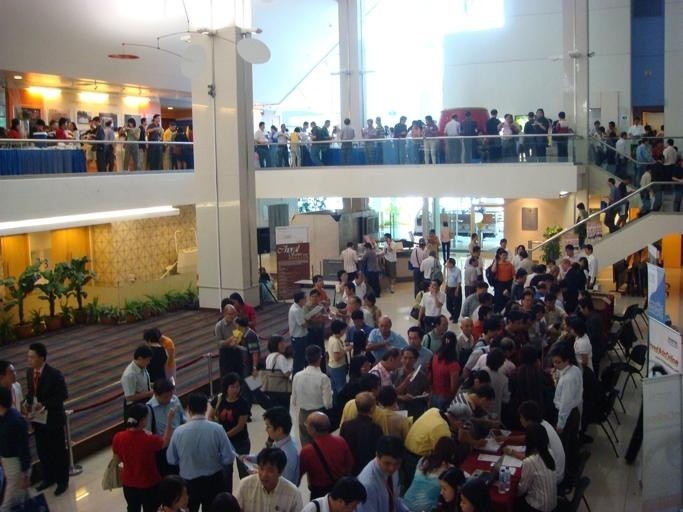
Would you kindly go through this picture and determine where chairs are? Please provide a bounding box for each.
[563,304,648,509]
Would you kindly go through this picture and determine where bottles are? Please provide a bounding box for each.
[498,465,511,493]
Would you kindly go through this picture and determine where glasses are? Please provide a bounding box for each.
[303,420,311,430]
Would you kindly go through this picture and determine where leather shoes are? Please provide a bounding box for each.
[37,479,55,492]
[54,483,67,495]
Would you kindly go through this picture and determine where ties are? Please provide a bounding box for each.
[32,368,40,395]
[386,476,395,512]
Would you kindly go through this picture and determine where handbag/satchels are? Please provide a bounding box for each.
[409,307,420,320]
[260,353,293,394]
[485,263,498,287]
[101,456,123,491]
[429,267,443,282]
[9,488,49,512]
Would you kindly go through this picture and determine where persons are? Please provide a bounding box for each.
[0,358,27,421]
[587,117,680,181]
[253,109,575,168]
[108,174,680,512]
[8,110,194,174]
[0,386,32,512]
[20,342,70,497]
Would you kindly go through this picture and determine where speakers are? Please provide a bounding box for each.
[257,227,270,254]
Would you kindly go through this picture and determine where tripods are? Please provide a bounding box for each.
[255,253,279,310]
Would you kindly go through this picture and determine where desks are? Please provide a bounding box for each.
[0,147,88,176]
[463,416,528,511]
